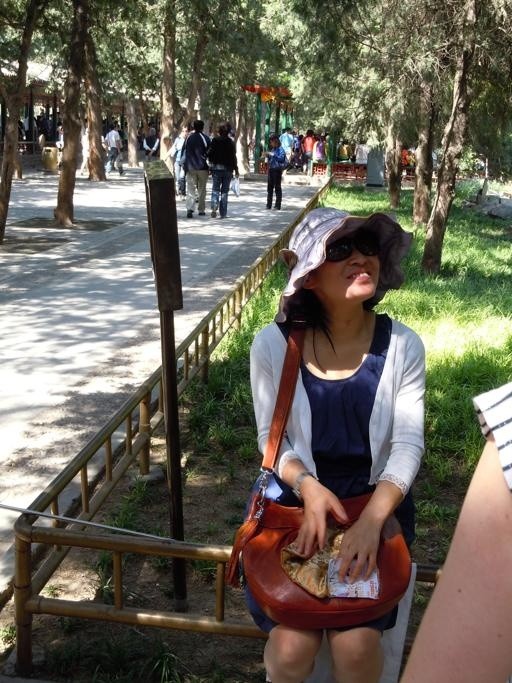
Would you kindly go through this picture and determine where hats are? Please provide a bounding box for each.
[274,206,413,324]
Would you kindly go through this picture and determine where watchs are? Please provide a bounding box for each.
[292,471,317,502]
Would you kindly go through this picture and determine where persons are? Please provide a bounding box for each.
[16,108,415,216]
[397,381,512,683]
[245,207,426,683]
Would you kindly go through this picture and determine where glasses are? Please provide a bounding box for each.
[326,232,380,263]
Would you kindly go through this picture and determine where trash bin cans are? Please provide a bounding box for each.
[41,147,57,173]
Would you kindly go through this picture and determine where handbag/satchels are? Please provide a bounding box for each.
[241,491,412,630]
[179,149,186,166]
[276,157,289,171]
